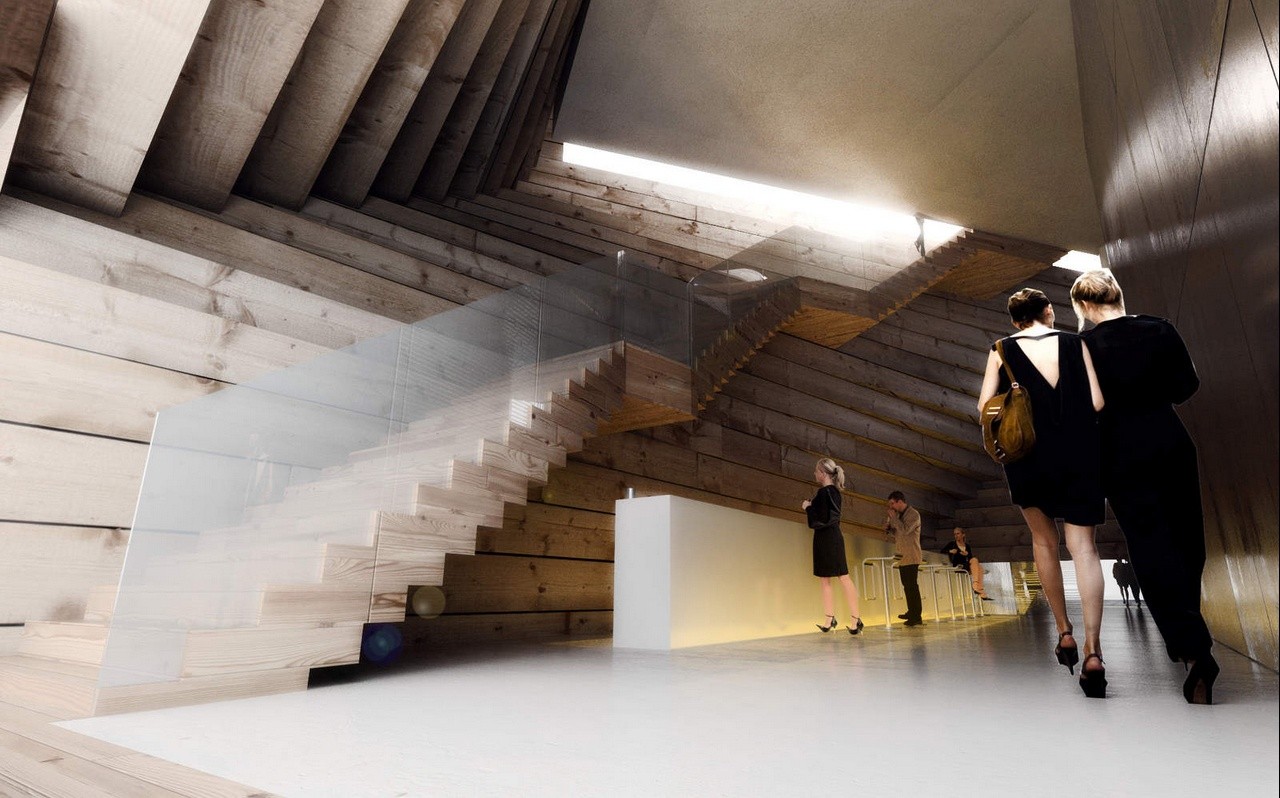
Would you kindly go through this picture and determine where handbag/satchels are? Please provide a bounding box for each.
[807,489,842,529]
[980,340,1037,467]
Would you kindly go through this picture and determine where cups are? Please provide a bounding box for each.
[627,488,635,499]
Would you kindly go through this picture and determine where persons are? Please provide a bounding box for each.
[976,287,1107,700]
[884,489,923,625]
[800,457,864,636]
[1064,267,1220,704]
[947,526,995,601]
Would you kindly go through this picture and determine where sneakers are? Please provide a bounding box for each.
[903,615,922,625]
[898,610,912,618]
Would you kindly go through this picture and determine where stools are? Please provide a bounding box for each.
[890,558,930,625]
[954,566,990,620]
[860,554,903,628]
[917,560,949,624]
[939,561,962,622]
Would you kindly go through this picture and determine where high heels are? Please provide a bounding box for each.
[816,615,837,635]
[1079,654,1108,698]
[1053,632,1079,676]
[979,592,994,601]
[972,581,983,595]
[1182,654,1221,706]
[846,616,864,637]
[1165,641,1191,673]
